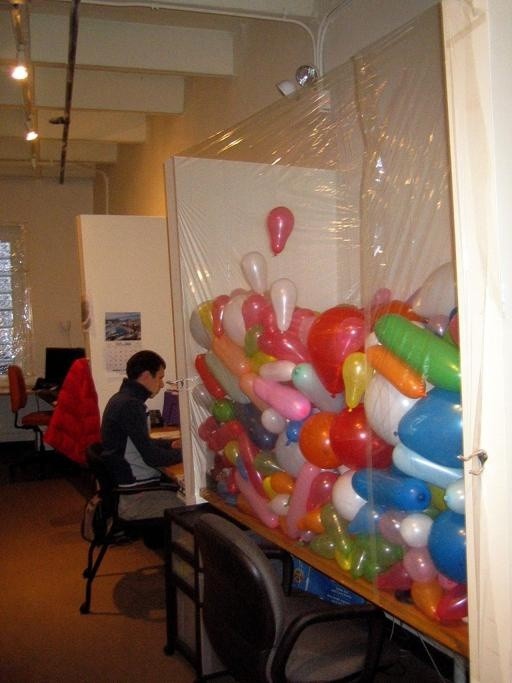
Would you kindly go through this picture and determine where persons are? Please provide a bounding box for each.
[100,350,182,548]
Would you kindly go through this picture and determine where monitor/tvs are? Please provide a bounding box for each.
[46,347,86,390]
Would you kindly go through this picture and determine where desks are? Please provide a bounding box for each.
[199,488,469,681]
[149,426,184,487]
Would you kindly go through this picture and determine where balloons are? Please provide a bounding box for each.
[269,206,294,255]
[188,253,467,629]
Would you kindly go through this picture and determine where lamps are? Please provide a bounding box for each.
[9,0,38,171]
[277,64,319,97]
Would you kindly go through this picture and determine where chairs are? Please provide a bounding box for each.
[195,513,386,683]
[80,444,185,614]
[9,366,53,480]
